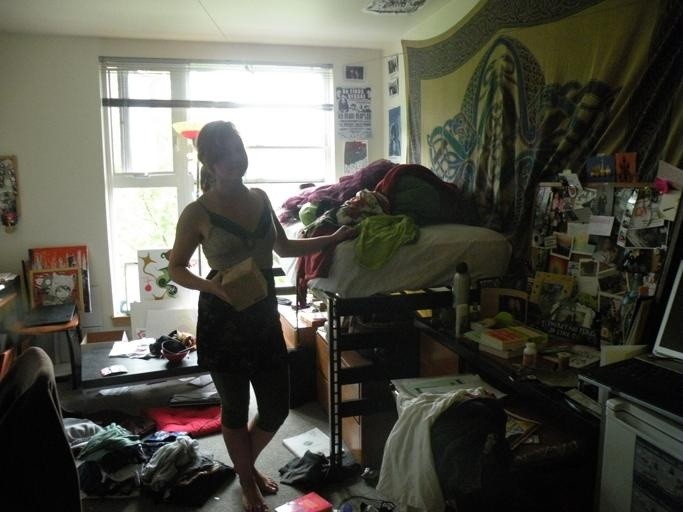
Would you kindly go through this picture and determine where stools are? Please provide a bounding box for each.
[3,312,82,390]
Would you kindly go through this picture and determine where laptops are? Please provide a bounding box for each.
[578,260,683,424]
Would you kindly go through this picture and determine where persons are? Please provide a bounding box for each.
[50,284,71,304]
[335,87,370,113]
[165,119,358,511]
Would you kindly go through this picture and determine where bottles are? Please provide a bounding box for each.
[522,342,536,367]
[452,261,470,308]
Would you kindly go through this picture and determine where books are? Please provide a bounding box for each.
[469,295,655,374]
[280,427,345,458]
[168,392,222,406]
[274,491,332,512]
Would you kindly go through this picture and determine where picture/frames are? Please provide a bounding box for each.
[21,244,91,314]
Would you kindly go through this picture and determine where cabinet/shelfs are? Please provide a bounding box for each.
[277,287,600,512]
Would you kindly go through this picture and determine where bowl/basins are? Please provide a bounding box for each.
[160,340,187,362]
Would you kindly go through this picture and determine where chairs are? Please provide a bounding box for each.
[0,347,82,512]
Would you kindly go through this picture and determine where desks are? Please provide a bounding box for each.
[80,341,209,390]
